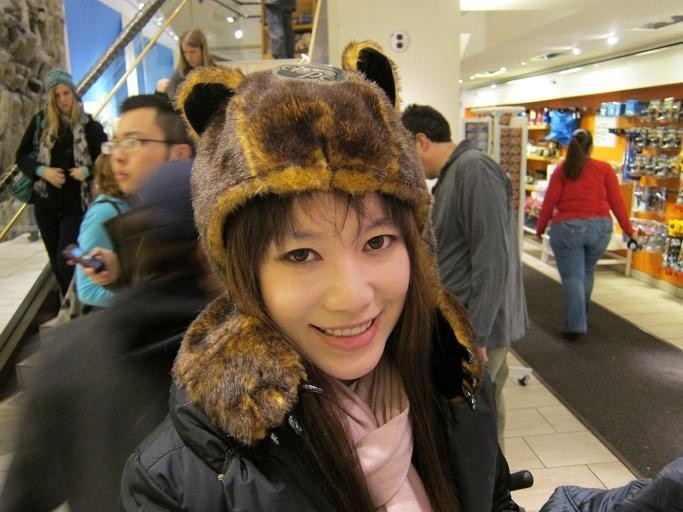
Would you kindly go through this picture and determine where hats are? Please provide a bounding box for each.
[192,66,434,264]
[45,68,75,93]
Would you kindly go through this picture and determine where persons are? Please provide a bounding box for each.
[64,92,205,295]
[116,41,521,510]
[73,145,140,309]
[14,66,110,313]
[151,26,235,95]
[398,103,533,459]
[533,127,646,341]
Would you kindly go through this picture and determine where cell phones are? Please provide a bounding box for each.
[63,244,104,274]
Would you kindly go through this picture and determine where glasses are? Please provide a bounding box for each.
[100,137,180,155]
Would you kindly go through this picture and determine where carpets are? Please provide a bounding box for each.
[509,260,683,482]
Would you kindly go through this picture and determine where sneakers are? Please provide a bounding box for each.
[560,329,585,339]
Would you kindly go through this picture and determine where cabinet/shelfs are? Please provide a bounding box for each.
[525,119,557,263]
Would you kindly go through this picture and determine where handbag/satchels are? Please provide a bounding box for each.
[8,172,43,205]
